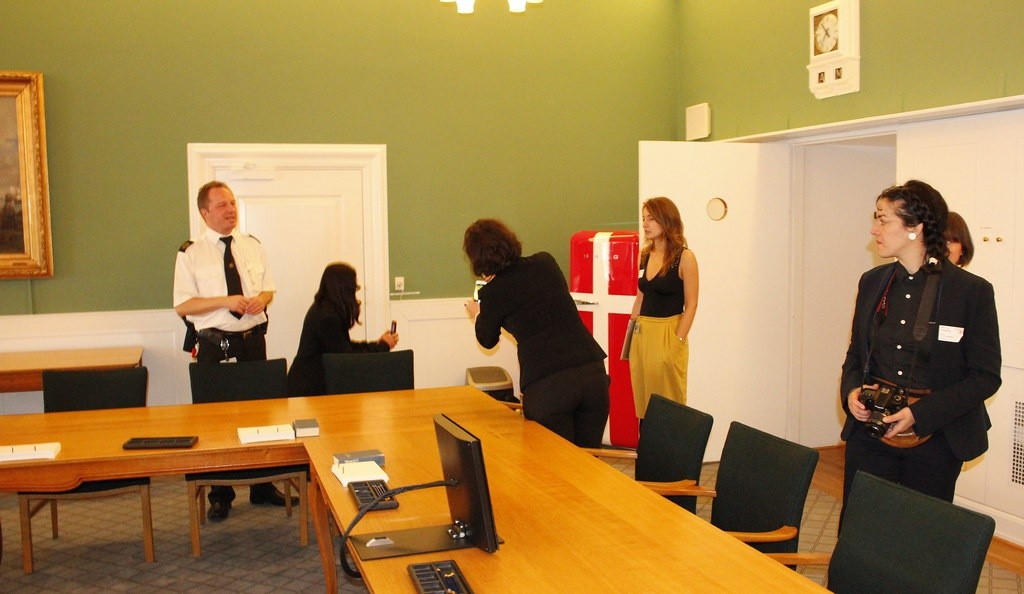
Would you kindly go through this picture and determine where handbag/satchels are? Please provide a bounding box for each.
[879,389,934,448]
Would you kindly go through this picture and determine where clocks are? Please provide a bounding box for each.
[806,0,860,101]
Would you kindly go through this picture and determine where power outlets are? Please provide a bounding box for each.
[395,277,405,291]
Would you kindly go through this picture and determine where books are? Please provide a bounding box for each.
[294,419,320,437]
[334,449,385,467]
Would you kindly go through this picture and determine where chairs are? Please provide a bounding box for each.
[322,348,415,396]
[763,468,997,594]
[581,393,714,516]
[16,366,155,575]
[184,358,309,559]
[645,420,820,573]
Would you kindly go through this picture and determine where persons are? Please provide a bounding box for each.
[289,262,399,396]
[629,197,699,436]
[173,182,298,523]
[464,219,610,447]
[837,180,1003,538]
[945,212,974,268]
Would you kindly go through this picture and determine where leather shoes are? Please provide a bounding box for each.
[251,484,300,506]
[207,501,232,521]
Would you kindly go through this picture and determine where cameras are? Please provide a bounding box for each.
[860,384,908,438]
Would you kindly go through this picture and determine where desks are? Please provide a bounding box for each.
[0,383,528,574]
[302,404,835,594]
[0,344,145,394]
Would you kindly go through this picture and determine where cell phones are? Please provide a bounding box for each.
[474,280,489,301]
[390,322,397,334]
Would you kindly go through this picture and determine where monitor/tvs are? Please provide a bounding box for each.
[432,413,500,553]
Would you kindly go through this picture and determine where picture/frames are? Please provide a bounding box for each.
[0,69,54,281]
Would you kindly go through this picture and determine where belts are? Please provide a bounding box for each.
[222,324,266,341]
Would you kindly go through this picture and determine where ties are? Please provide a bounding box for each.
[219,235,245,320]
